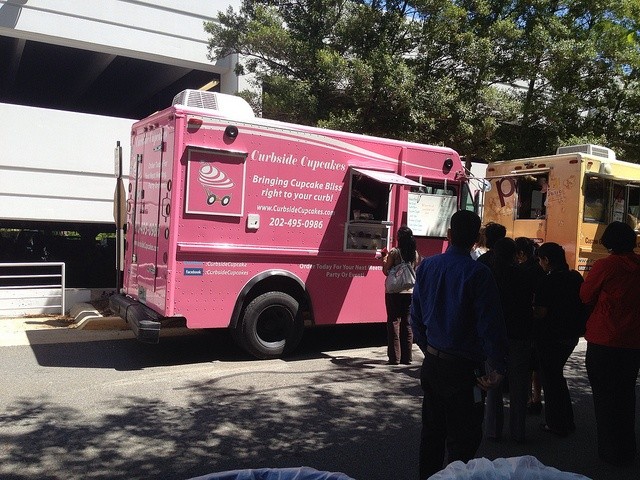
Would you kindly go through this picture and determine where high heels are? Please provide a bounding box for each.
[527,405,536,415]
[536,401,543,414]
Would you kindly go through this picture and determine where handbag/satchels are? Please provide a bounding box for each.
[386,247,416,293]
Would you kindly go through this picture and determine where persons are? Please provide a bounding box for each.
[516,238,544,413]
[524,243,589,436]
[485,239,531,443]
[382,226,421,363]
[579,221,640,462]
[477,222,507,273]
[410,211,509,480]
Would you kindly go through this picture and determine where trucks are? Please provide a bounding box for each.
[109,88,472,360]
[479,145,640,282]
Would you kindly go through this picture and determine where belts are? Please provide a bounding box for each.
[426,344,474,367]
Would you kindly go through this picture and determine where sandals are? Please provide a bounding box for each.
[539,424,550,432]
